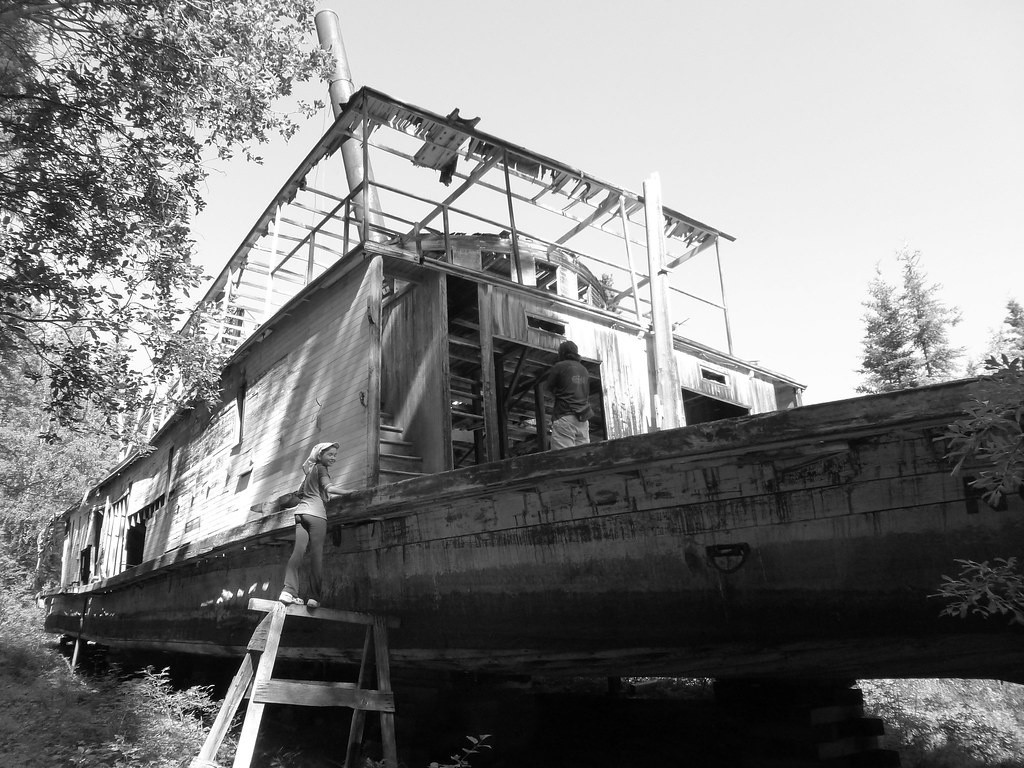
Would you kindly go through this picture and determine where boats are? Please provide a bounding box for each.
[38,9,1024,686]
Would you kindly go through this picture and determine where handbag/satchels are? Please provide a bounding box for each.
[575,405,595,422]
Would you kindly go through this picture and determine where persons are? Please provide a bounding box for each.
[279,441,340,610]
[547,340,593,452]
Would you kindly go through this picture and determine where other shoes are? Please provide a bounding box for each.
[307,599,319,610]
[279,592,305,606]
